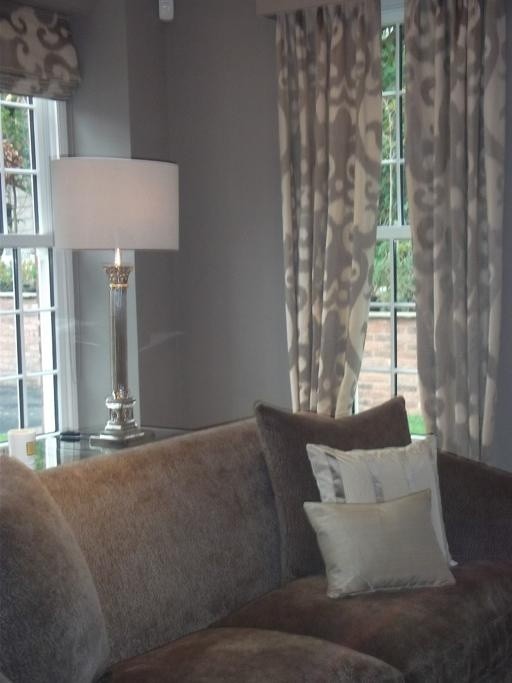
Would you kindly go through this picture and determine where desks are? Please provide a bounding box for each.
[1,419,181,471]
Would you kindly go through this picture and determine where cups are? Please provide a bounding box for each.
[9,428,38,466]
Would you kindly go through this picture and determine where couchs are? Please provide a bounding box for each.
[1,401,511,683]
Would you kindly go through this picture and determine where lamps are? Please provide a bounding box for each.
[47,153,183,447]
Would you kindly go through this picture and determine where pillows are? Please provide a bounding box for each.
[249,387,415,594]
[306,428,463,569]
[299,488,457,604]
[0,449,114,683]
